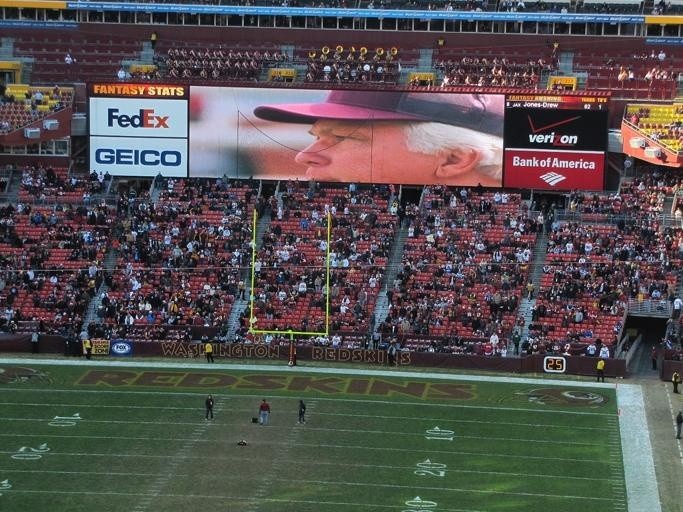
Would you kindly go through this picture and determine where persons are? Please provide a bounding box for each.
[259,398,271,427]
[205,394,215,422]
[299,399,306,423]
[671,369,681,393]
[675,409,683,439]
[596,358,605,383]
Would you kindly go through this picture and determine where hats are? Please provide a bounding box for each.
[254,89,506,138]
